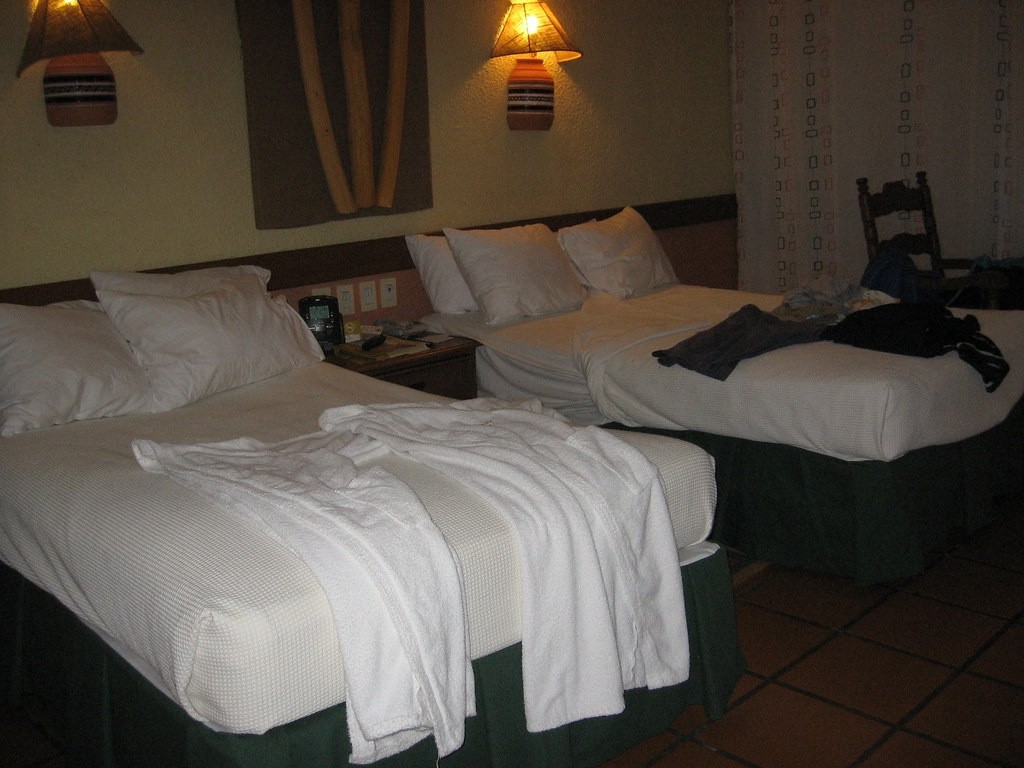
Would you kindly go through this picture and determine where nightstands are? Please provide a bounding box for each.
[320,319,483,401]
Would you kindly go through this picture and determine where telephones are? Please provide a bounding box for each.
[374,315,429,339]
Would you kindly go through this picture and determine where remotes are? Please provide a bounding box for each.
[320,343,335,354]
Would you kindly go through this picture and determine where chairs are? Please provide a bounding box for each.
[854,173,1017,313]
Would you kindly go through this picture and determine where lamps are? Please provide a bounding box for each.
[15,0,145,128]
[490,0,585,132]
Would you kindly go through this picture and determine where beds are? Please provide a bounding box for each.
[299,195,1024,594]
[0,253,751,768]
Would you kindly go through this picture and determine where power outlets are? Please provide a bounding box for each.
[359,281,378,312]
[336,284,355,315]
[380,276,398,309]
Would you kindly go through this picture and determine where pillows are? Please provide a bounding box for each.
[96,275,324,410]
[557,205,678,298]
[442,224,593,326]
[403,233,482,316]
[0,299,165,438]
[89,265,272,299]
